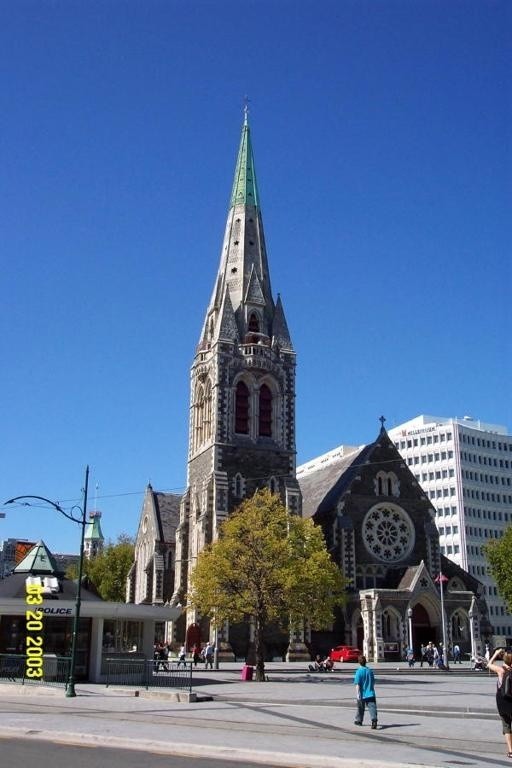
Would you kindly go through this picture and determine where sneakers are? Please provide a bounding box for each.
[353,718,379,729]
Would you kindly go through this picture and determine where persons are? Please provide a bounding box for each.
[313,654,335,673]
[404,640,463,672]
[151,639,215,674]
[486,648,512,758]
[352,655,378,729]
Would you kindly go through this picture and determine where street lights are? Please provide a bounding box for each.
[468,608,476,662]
[408,605,414,654]
[4,465,105,699]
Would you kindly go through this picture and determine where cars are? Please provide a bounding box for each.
[325,645,361,662]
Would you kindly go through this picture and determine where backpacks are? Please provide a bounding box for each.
[499,665,512,701]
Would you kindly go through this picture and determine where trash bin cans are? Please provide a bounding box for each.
[242,665,253,681]
